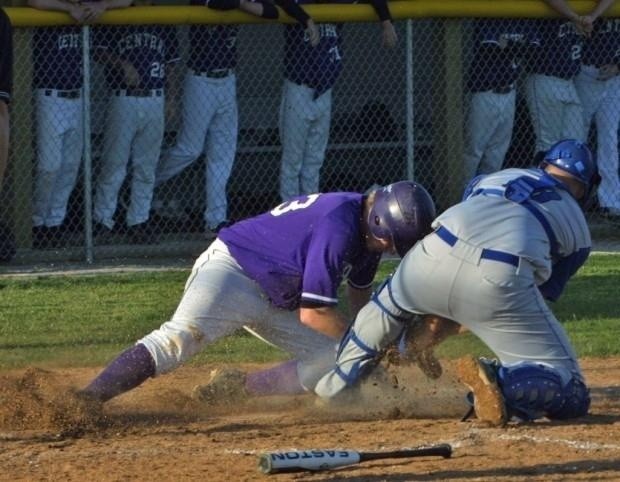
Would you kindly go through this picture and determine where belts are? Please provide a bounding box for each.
[194,70,229,78]
[45,90,80,99]
[115,89,162,96]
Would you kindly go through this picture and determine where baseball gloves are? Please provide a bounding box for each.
[386,336,443,381]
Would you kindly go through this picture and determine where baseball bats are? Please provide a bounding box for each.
[256,444,452,473]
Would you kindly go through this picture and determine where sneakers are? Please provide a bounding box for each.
[190,367,245,405]
[65,389,104,416]
[456,354,512,428]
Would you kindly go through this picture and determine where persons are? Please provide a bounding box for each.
[75,180,435,402]
[463,2,619,225]
[314,137,598,427]
[2,0,397,246]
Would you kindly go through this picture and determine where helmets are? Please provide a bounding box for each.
[367,180,437,260]
[543,139,596,185]
